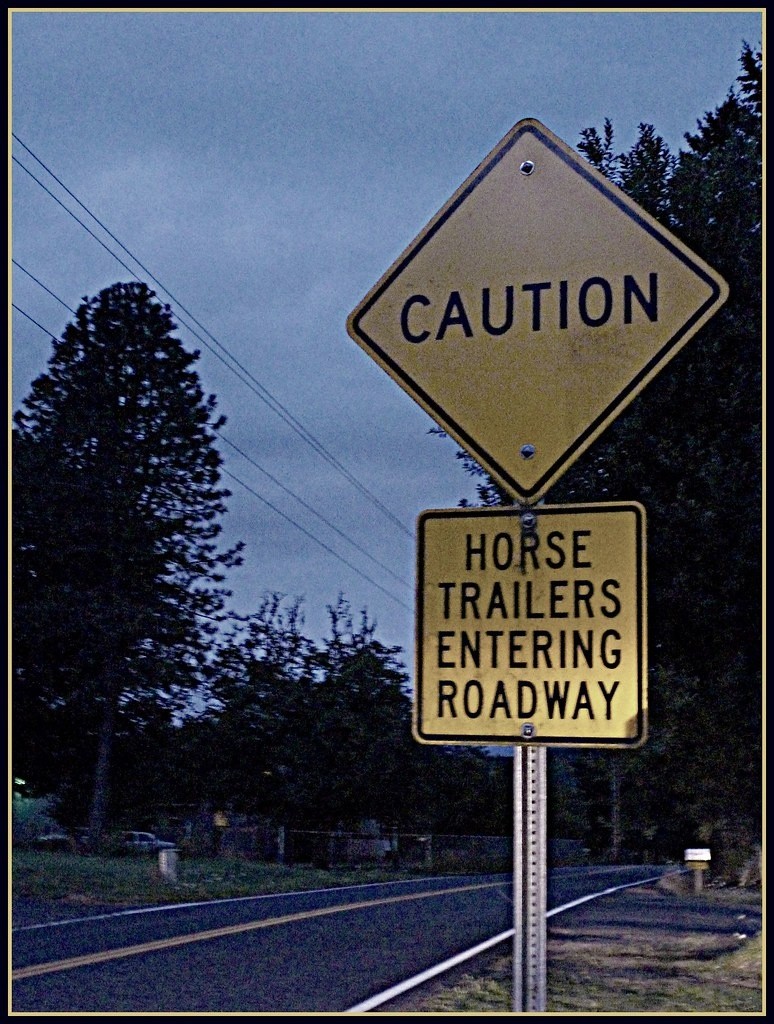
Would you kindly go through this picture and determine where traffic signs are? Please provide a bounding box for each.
[344,117,732,504]
[411,506,650,750]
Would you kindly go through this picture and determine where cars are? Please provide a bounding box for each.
[37,830,176,859]
